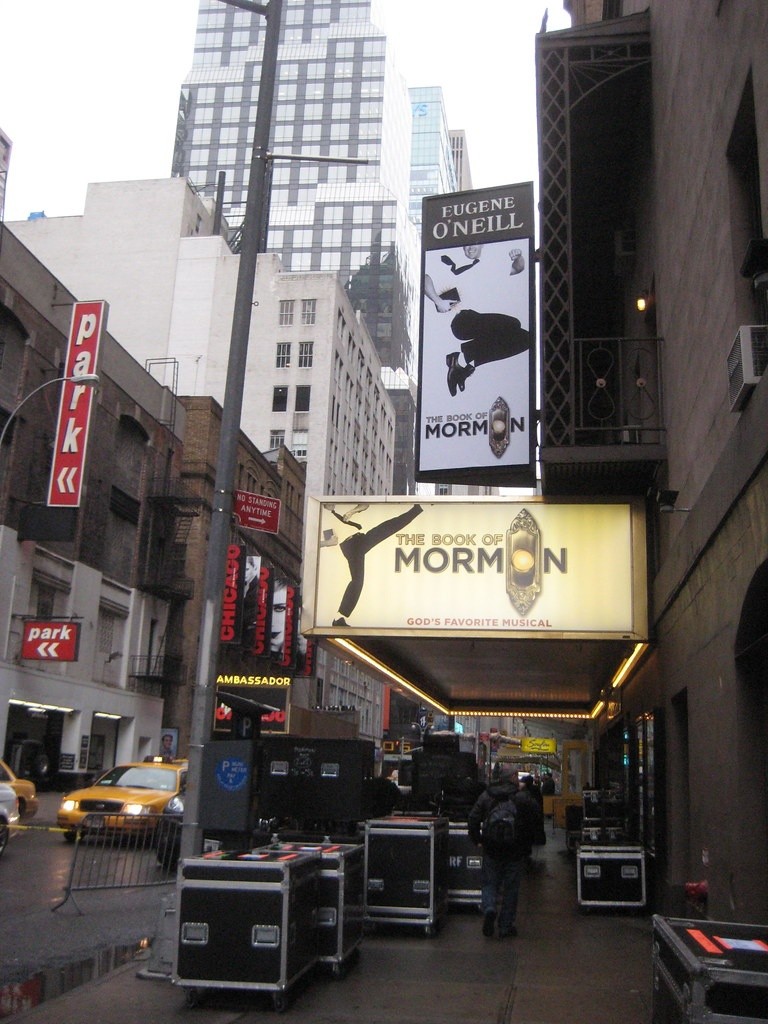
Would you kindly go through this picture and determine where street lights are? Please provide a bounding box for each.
[412,722,434,748]
[396,738,404,777]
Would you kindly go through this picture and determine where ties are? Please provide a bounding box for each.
[441,255,479,275]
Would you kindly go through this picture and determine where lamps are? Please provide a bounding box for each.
[103,651,123,664]
[636,289,649,311]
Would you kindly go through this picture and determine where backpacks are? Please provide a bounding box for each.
[481,800,518,861]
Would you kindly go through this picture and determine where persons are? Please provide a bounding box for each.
[469,764,538,938]
[320,502,430,628]
[242,556,259,646]
[269,576,288,661]
[422,244,529,395]
[159,735,176,763]
[522,772,555,846]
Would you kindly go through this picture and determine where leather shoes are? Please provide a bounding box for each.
[459,364,474,393]
[445,352,462,397]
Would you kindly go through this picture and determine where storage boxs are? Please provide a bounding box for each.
[649,913,768,1024]
[574,788,648,915]
[168,804,486,1011]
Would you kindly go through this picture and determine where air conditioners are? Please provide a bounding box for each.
[726,324,768,415]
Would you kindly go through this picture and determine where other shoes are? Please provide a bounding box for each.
[482,910,497,937]
[498,926,518,938]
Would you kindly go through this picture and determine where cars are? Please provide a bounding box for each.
[157,781,186,871]
[57,755,189,841]
[0,786,21,854]
[0,759,41,826]
[518,771,530,782]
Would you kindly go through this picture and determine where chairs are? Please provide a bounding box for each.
[564,804,585,851]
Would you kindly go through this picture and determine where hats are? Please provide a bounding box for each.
[517,775,533,784]
[492,758,518,778]
